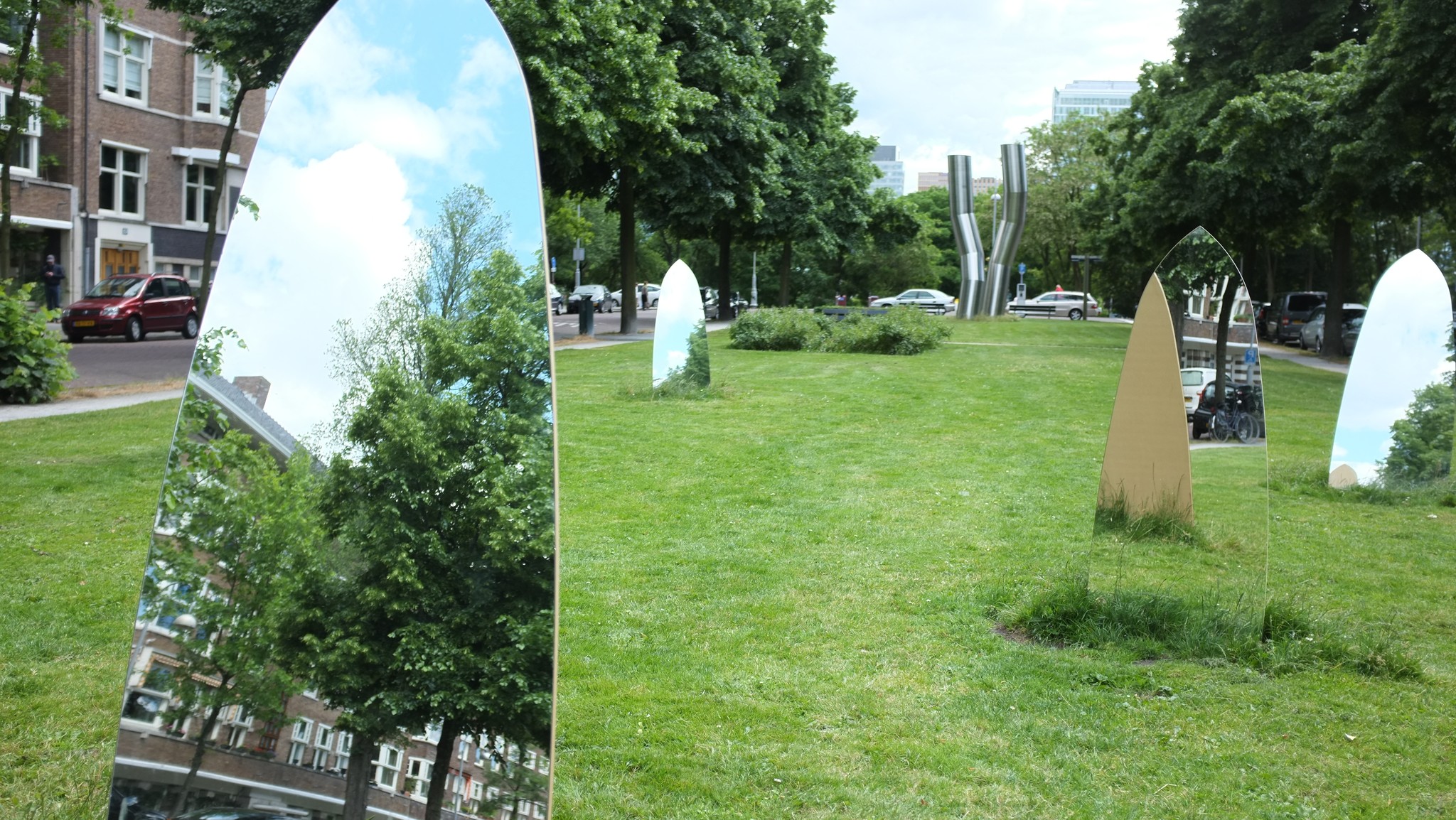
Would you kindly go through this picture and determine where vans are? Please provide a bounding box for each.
[1266,292,1328,349]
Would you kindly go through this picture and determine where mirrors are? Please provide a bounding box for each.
[108,0,560,820]
[652,258,711,388]
[1079,224,1271,644]
[1326,248,1456,490]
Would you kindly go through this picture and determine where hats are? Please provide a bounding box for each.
[46,254,55,262]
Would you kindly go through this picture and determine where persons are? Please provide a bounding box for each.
[1055,284,1064,300]
[635,282,639,308]
[39,252,67,324]
[837,294,846,307]
[641,281,649,311]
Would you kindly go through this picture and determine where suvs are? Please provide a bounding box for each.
[698,286,719,318]
[1189,378,1262,439]
[1180,367,1236,421]
[61,271,199,340]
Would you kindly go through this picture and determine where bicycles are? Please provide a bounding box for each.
[1206,391,1262,444]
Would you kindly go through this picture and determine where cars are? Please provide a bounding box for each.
[609,282,661,311]
[1298,302,1369,355]
[871,288,956,317]
[568,285,612,313]
[727,290,749,315]
[1251,300,1271,333]
[1006,290,1100,321]
[548,283,562,316]
